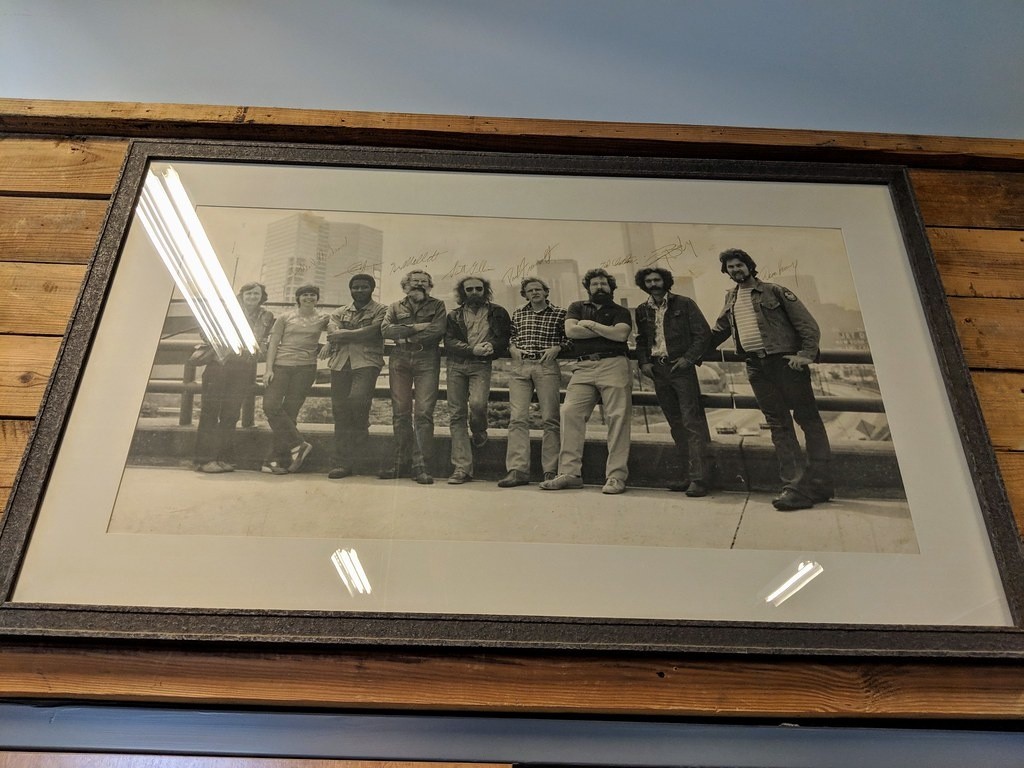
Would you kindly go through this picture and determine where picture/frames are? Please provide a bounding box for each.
[0,138,1024,660]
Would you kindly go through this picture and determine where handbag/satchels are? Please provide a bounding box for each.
[189,344,214,366]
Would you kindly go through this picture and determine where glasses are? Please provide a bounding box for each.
[464,286,484,292]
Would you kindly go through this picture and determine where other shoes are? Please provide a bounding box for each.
[772,487,826,509]
[414,465,432,484]
[261,458,287,475]
[545,471,554,481]
[448,467,471,483]
[540,474,584,490]
[497,468,529,487]
[667,476,692,490]
[195,460,233,473]
[355,448,372,474]
[602,477,626,494]
[377,464,409,479]
[472,429,488,446]
[329,460,352,478]
[687,481,705,497]
[290,442,313,472]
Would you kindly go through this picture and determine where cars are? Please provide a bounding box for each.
[716,419,738,434]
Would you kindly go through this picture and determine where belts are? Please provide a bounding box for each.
[522,353,543,360]
[650,356,669,363]
[395,342,434,351]
[746,350,768,358]
[577,351,620,362]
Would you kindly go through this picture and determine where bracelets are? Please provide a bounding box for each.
[405,337,408,343]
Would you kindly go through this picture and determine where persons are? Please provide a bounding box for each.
[378,270,448,484]
[262,285,331,474]
[327,274,390,479]
[697,248,834,510]
[498,277,574,487]
[539,269,632,494]
[443,277,511,484]
[635,268,713,496]
[194,282,274,473]
